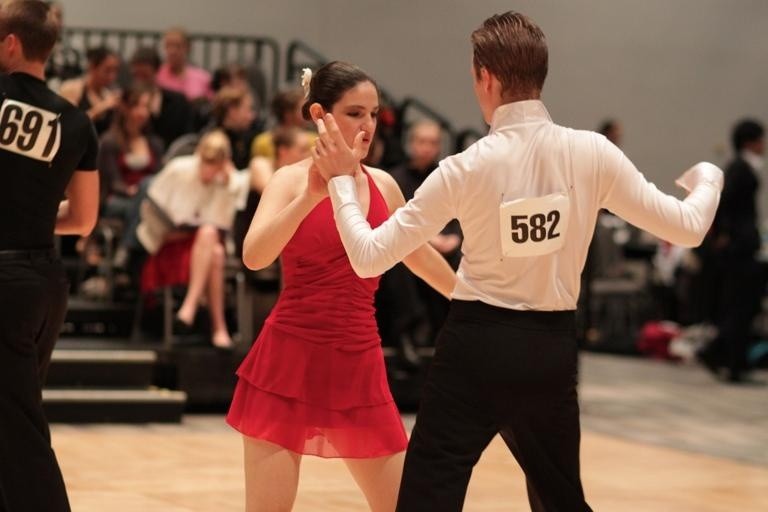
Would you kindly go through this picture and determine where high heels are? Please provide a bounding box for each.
[212,342,236,353]
[173,319,197,337]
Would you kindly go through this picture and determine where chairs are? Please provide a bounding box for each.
[132,176,253,351]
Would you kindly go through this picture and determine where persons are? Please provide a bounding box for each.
[310,9,725,512]
[0,0,101,512]
[360,99,490,372]
[46,31,319,354]
[651,238,704,326]
[577,216,603,351]
[597,119,620,146]
[226,60,457,512]
[694,121,768,384]
